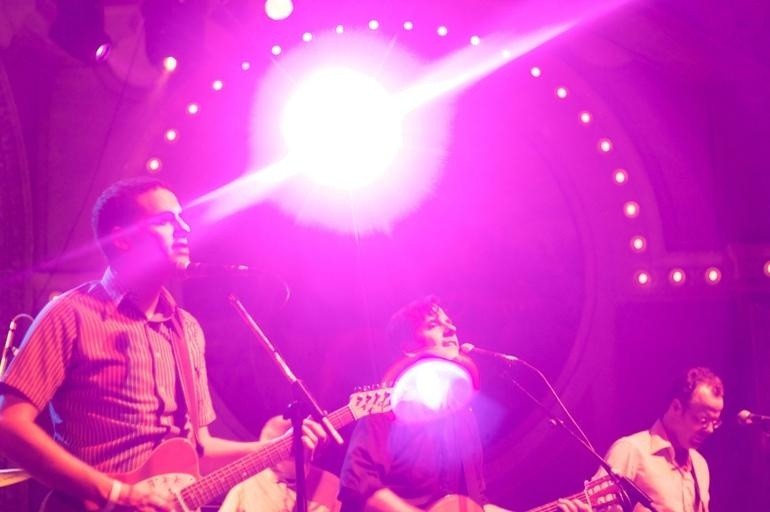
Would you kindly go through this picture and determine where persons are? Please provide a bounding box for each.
[338,293,591,512]
[580,365,727,512]
[218,413,343,512]
[0,174,329,511]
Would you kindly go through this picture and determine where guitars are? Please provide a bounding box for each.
[424,474,627,511]
[41,382,404,512]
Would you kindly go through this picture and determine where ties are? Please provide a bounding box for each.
[670,458,694,512]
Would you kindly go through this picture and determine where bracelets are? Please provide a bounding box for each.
[102,478,123,511]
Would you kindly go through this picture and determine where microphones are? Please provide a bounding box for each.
[460,343,505,362]
[735,409,770,426]
[167,255,237,281]
